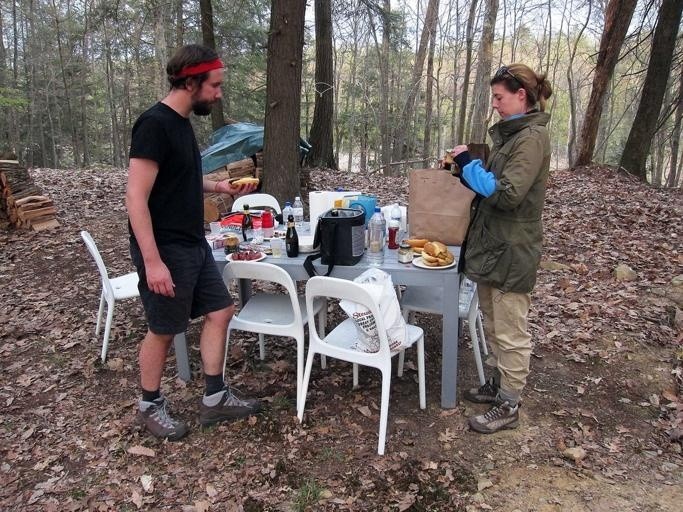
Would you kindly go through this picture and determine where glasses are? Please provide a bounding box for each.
[495,66,521,85]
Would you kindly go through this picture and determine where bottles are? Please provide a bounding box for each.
[397,244,412,264]
[285,215,299,258]
[388,220,399,249]
[242,196,304,238]
[391,202,401,221]
[270,232,284,258]
[368,208,384,266]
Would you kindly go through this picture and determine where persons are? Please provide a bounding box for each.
[122,43,264,443]
[436,62,556,434]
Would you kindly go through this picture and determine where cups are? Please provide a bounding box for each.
[399,223,409,241]
[225,238,239,254]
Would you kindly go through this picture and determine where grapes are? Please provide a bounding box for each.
[233,251,260,261]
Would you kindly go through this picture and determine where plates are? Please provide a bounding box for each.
[413,251,422,256]
[225,251,267,262]
[414,256,456,269]
[252,245,273,254]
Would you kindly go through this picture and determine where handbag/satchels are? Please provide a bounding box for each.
[313,207,365,266]
[338,268,409,353]
[408,167,475,246]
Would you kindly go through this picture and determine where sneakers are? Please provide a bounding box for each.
[469,400,520,434]
[463,376,523,408]
[199,385,260,424]
[138,394,188,442]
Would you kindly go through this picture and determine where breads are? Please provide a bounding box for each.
[232,176,260,189]
[421,241,454,267]
[401,239,430,253]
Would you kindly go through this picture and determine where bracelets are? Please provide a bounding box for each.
[214,180,222,194]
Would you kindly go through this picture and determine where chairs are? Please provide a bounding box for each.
[78,193,489,455]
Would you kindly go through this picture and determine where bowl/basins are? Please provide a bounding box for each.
[299,236,320,253]
